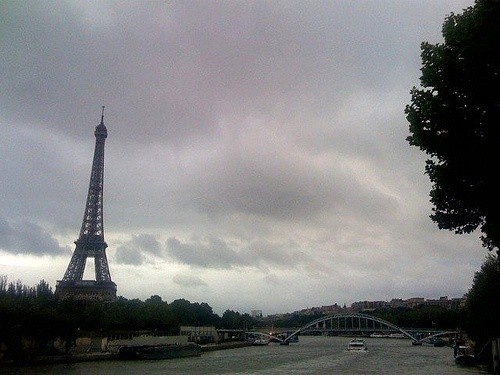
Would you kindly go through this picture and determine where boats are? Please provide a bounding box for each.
[252,334,271,346]
[369,333,389,338]
[453,346,476,367]
[434,337,445,347]
[347,338,368,351]
[388,334,405,338]
[118,342,202,361]
[273,331,300,343]
